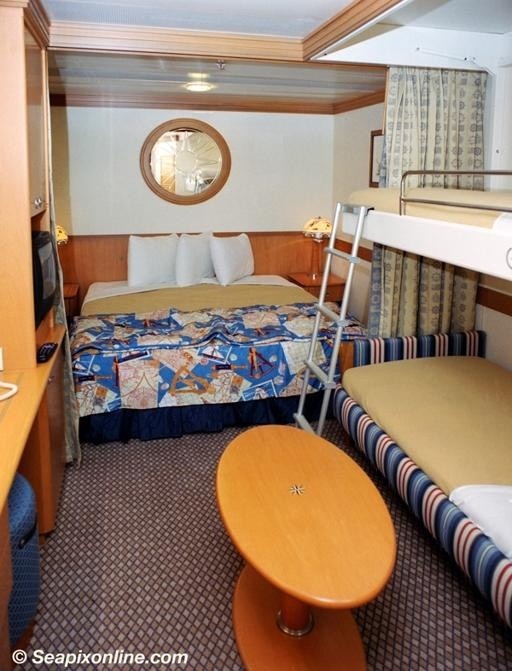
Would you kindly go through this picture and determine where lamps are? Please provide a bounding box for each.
[303,217,334,280]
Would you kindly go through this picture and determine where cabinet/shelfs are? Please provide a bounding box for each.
[0,0,67,534]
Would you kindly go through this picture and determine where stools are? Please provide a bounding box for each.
[5,471,41,650]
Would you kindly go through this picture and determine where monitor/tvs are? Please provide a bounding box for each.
[31,229,58,331]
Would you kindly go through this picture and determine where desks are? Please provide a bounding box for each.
[0,369,45,670]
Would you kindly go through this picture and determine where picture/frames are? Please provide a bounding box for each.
[369,128,385,187]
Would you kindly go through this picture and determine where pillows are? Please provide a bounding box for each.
[126,232,256,288]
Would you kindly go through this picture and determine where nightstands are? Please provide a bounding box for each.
[63,282,80,329]
[288,272,346,303]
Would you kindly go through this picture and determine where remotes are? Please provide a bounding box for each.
[37,342,58,363]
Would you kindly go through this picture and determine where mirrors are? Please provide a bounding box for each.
[139,117,231,207]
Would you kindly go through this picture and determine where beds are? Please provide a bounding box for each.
[71,274,368,442]
[290,168,512,628]
